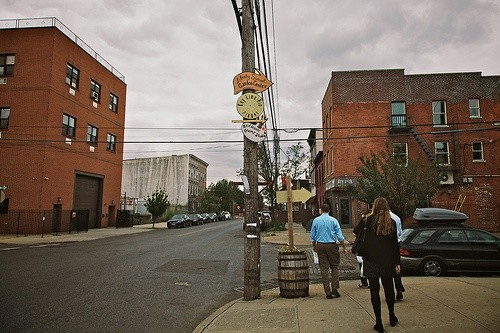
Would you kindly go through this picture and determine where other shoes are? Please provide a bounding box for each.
[332,289,340,297]
[396,292,403,299]
[326,295,332,299]
[390,316,398,327]
[374,324,384,333]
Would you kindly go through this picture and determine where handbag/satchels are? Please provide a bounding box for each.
[311,250,319,265]
[351,215,368,254]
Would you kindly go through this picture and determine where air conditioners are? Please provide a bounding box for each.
[436,171,454,184]
[91,92,98,98]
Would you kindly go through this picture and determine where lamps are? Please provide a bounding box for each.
[58,197,62,202]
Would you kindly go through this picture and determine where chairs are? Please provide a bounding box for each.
[445,232,453,241]
[458,233,465,240]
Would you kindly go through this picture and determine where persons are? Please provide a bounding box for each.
[353,210,372,288]
[310,204,346,298]
[388,210,405,301]
[353,197,401,333]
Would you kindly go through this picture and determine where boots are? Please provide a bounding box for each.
[358,275,368,287]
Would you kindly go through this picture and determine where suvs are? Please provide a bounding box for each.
[398,208,500,277]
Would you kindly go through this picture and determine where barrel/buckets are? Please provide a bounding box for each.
[277,251,310,298]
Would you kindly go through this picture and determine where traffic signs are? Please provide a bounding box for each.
[0,186,8,190]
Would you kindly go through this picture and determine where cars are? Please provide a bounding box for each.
[243,210,271,231]
[167,212,231,228]
